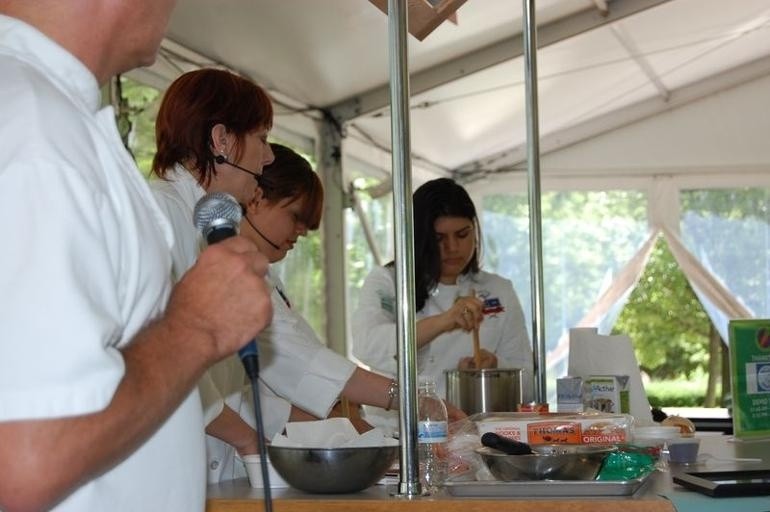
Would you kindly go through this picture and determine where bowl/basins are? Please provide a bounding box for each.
[631,426,682,453]
[667,436,701,464]
[474,441,618,483]
[242,452,293,491]
[265,443,400,496]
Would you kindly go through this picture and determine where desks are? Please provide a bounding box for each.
[206,435,770,512]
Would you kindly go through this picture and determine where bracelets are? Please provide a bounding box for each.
[385,379,399,411]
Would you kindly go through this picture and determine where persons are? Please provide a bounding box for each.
[349,175,536,413]
[228,138,475,479]
[1,0,275,512]
[144,66,376,482]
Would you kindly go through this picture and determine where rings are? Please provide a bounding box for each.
[462,307,473,315]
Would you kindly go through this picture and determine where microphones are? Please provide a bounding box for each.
[225,160,272,191]
[245,214,279,250]
[192,190,260,378]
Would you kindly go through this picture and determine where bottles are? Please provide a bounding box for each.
[417,379,450,497]
